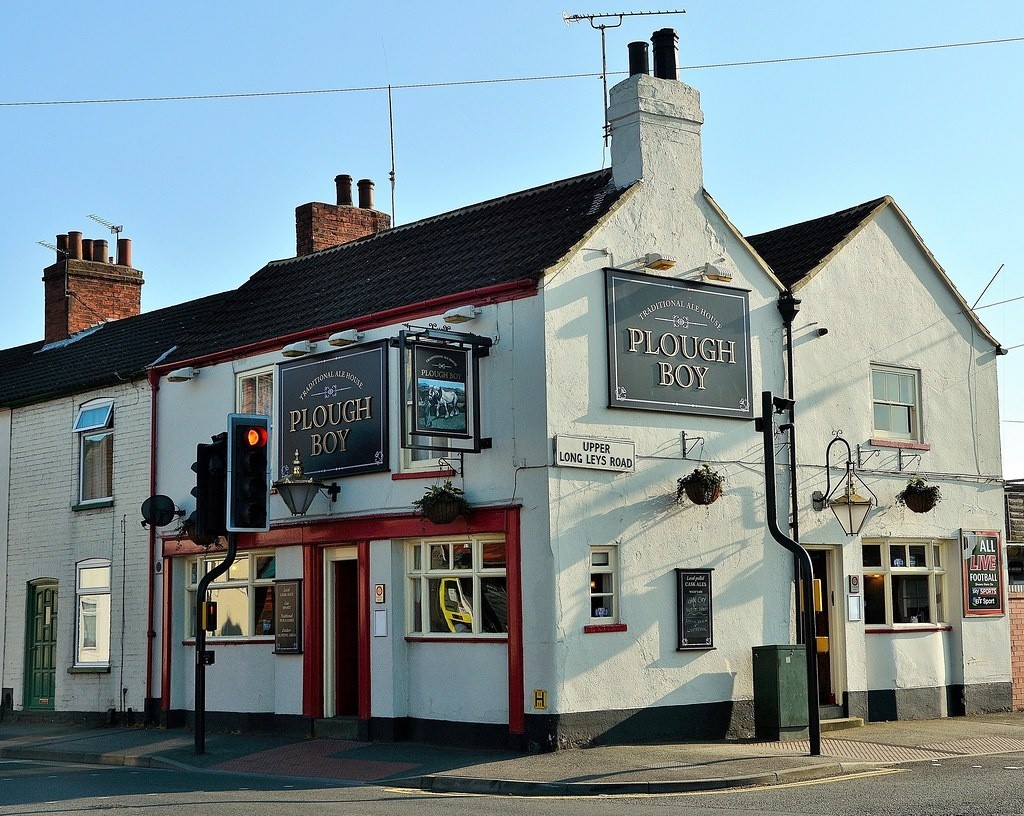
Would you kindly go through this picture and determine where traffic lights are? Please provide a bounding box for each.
[227,413,271,533]
[203,601,217,632]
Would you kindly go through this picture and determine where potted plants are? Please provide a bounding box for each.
[675,463,728,507]
[411,485,475,539]
[174,510,229,555]
[894,476,944,522]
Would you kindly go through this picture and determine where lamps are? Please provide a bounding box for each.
[328,328,366,346]
[282,340,316,356]
[699,262,733,282]
[272,450,341,517]
[167,366,200,383]
[811,428,878,537]
[639,254,677,270]
[443,305,483,323]
